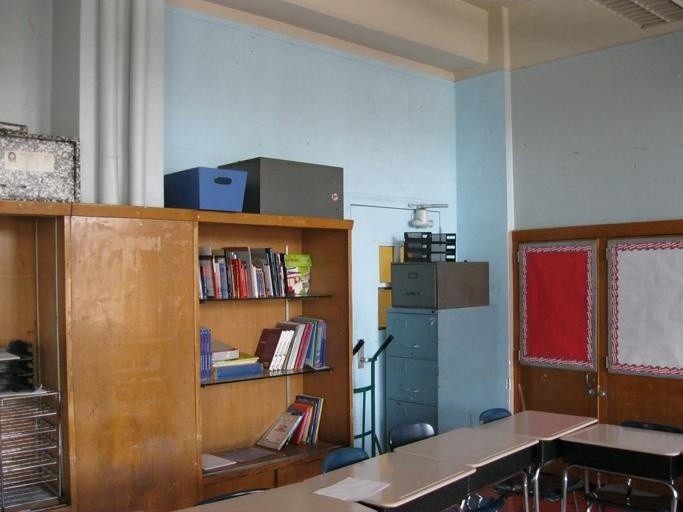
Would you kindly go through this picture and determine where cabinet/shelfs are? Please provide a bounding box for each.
[194,214,355,500]
[386,303,499,453]
[1,202,196,511]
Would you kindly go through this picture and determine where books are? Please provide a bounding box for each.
[200,453,237,472]
[198,247,303,299]
[256,394,324,451]
[200,316,327,385]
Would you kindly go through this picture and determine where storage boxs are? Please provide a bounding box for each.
[389,262,489,310]
[216,157,343,220]
[1,129,81,202]
[403,231,456,263]
[165,167,248,212]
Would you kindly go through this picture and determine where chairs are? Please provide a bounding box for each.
[583,422,682,512]
[389,421,507,511]
[322,447,369,474]
[479,408,583,511]
[193,489,269,507]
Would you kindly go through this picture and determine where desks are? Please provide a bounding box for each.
[393,427,539,510]
[559,424,682,512]
[481,410,598,512]
[305,452,477,512]
[174,480,377,512]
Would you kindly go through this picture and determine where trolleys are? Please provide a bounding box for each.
[352,333,395,457]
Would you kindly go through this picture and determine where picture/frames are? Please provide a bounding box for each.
[254,395,324,450]
